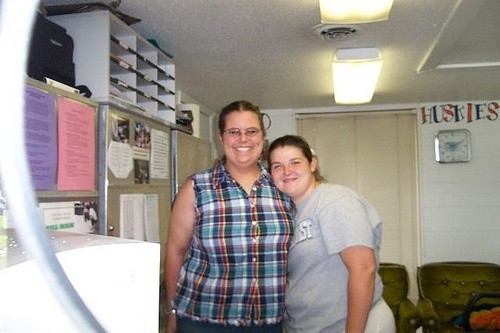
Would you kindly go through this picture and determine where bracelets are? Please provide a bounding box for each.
[166,308,176,316]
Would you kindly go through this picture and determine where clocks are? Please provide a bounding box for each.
[435,128,471,162]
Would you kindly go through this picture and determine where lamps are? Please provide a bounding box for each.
[320,0,394,24]
[332,47,381,106]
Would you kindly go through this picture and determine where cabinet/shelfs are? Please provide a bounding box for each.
[45,10,176,123]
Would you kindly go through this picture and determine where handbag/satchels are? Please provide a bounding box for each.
[27,11,91,98]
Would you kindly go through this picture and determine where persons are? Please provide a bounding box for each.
[268,135,396,333]
[112,123,151,181]
[164,101,295,333]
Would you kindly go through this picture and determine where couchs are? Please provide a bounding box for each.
[378,261,500,333]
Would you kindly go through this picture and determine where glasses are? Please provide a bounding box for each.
[223,127,261,137]
